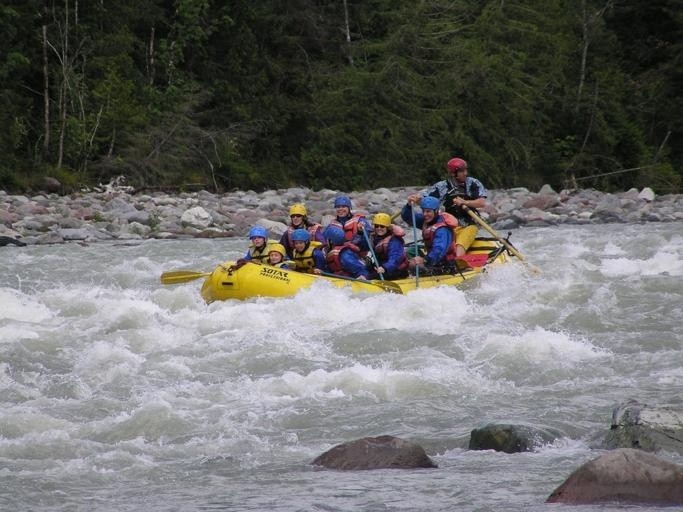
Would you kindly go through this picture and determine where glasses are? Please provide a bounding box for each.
[291,215,302,218]
[375,225,386,228]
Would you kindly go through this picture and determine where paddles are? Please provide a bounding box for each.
[456,258,471,269]
[160,270,213,284]
[319,270,402,294]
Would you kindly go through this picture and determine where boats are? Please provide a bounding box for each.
[198,234,512,308]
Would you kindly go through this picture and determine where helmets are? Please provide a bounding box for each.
[419,197,441,210]
[334,196,351,208]
[447,158,467,172]
[372,213,391,227]
[322,226,345,245]
[291,229,310,243]
[268,244,286,257]
[250,226,268,242]
[289,204,307,217]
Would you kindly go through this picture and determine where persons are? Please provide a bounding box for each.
[237,195,458,282]
[415,157,488,229]
